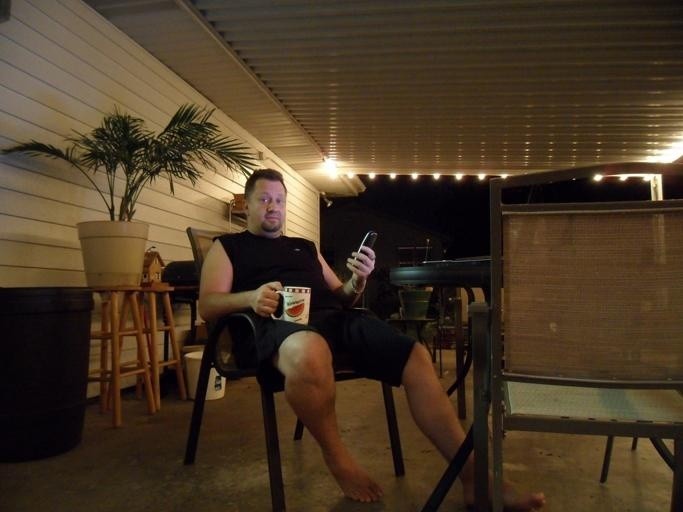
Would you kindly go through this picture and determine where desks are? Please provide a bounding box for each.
[389,264,490,512]
[386,319,435,357]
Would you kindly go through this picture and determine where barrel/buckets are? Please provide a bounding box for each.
[0,286,96,466]
[184,350,231,400]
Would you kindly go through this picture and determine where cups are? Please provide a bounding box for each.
[271,286,312,328]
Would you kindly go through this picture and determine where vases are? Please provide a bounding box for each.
[398,289,432,321]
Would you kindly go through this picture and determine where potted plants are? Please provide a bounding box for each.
[0,103,263,288]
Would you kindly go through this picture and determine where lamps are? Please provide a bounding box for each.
[321,191,333,208]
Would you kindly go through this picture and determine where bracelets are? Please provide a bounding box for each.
[351,277,367,295]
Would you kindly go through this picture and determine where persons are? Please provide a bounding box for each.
[198,168,546,512]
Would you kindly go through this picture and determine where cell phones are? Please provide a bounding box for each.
[352,230,378,267]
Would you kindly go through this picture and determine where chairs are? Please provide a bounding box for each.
[182,228,405,512]
[470,162,682,512]
[454,286,487,420]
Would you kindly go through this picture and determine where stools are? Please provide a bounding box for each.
[107,287,187,412]
[88,284,156,429]
